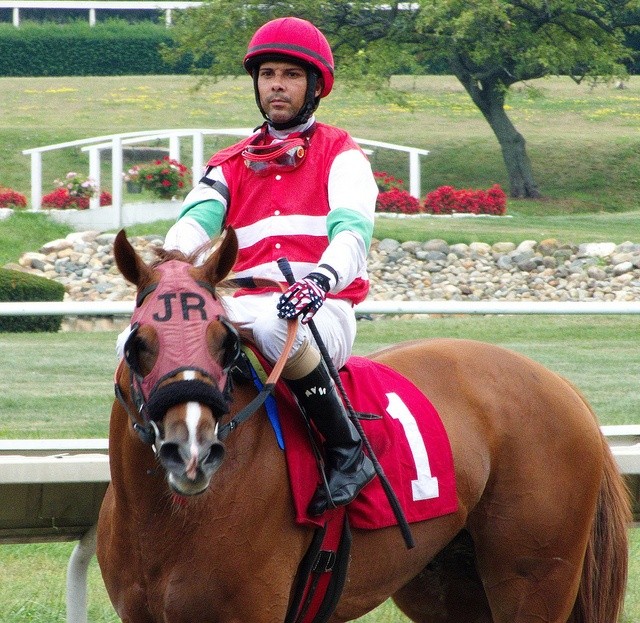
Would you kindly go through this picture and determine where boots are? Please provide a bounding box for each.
[282,351,377,518]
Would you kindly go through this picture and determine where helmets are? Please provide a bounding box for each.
[244,16,335,99]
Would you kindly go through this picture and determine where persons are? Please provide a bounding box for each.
[162,16,380,519]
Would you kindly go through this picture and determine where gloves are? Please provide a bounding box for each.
[277,271,330,325]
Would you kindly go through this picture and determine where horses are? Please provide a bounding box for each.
[93,218,640,623]
